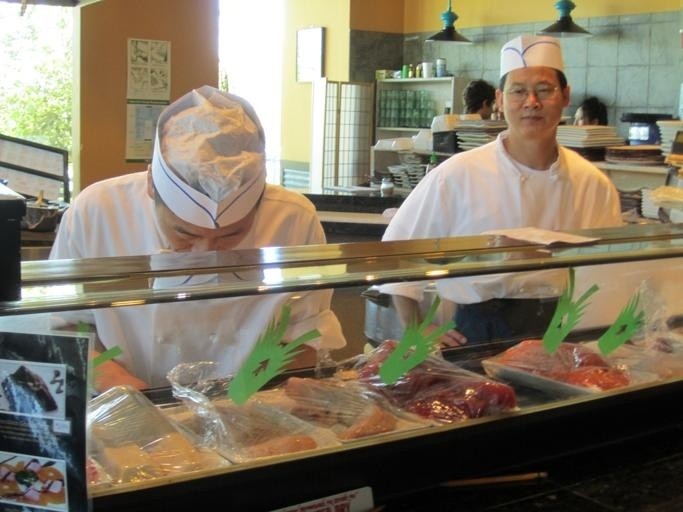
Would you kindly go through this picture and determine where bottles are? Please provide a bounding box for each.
[381,178,394,195]
[408,64,414,78]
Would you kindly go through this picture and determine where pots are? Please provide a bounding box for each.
[23,204,59,232]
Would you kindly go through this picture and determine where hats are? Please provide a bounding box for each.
[151,86,265,230]
[500,36,564,80]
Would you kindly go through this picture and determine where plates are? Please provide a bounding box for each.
[453,118,683,168]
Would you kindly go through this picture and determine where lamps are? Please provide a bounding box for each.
[423,1,595,45]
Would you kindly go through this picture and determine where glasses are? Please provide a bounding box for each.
[502,86,559,100]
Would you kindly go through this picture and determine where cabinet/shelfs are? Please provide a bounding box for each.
[374,76,469,141]
[365,146,677,221]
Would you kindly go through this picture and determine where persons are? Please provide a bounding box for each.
[573,94,609,127]
[461,79,496,122]
[36,87,349,397]
[379,36,623,349]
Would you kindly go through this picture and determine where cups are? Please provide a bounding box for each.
[423,62,433,78]
